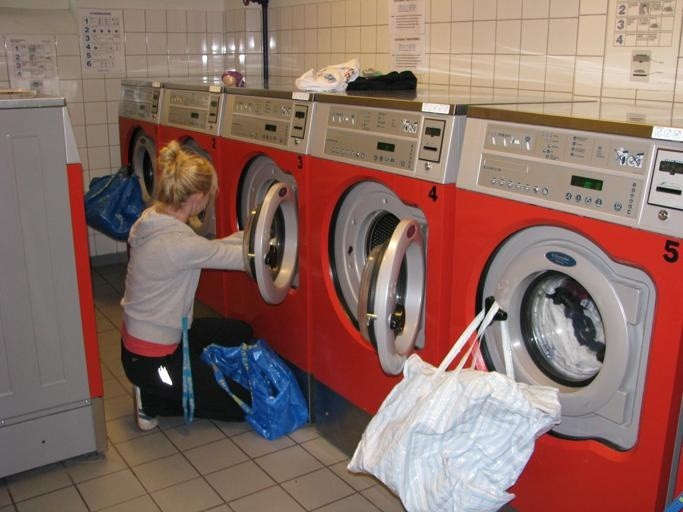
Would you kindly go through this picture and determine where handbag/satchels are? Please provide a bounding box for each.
[83,165,143,240]
[346,352,562,512]
[198,337,310,441]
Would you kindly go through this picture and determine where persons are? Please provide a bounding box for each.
[121,139,247,431]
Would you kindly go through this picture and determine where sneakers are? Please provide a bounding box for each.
[132,385,158,432]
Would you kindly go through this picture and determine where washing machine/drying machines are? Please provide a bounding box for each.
[0,89,107,480]
[117,72,683,512]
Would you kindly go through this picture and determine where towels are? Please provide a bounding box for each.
[294,57,418,97]
